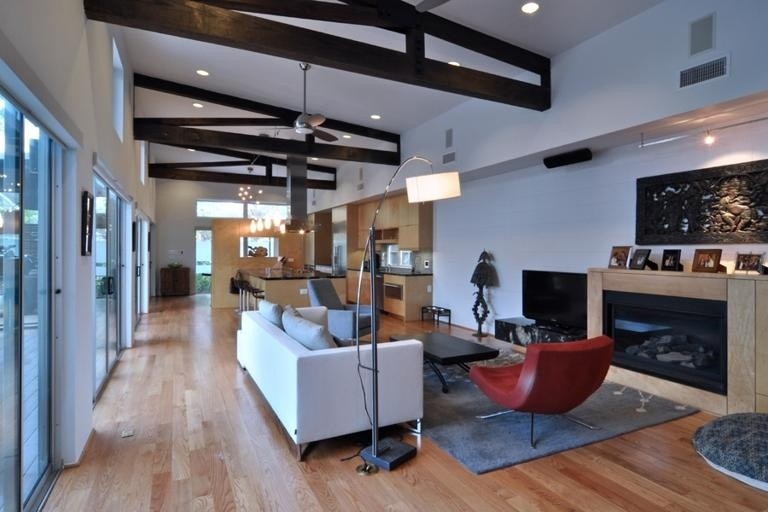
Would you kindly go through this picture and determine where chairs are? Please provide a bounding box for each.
[306,278,380,347]
[468,335,615,451]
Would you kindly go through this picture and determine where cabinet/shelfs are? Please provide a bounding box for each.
[495,317,587,354]
[348,196,433,322]
[160,266,190,297]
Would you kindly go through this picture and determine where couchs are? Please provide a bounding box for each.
[239,306,424,461]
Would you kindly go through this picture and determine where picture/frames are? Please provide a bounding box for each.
[661,249,683,271]
[607,246,633,269]
[691,248,727,274]
[133,222,136,252]
[733,251,766,276]
[81,190,94,256]
[630,249,658,270]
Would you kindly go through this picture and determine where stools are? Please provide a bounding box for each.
[234,272,265,317]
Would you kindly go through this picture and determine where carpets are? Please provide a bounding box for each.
[411,348,701,475]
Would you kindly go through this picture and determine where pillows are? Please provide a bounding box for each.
[283,304,305,319]
[281,310,338,349]
[257,298,285,331]
[690,412,767,493]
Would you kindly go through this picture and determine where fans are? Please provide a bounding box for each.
[264,62,339,142]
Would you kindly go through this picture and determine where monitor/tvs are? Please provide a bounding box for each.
[521,269,588,328]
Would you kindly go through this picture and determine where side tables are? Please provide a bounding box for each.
[422,305,451,327]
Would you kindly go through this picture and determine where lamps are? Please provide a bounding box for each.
[356,156,463,473]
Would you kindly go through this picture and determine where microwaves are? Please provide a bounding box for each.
[401,251,412,267]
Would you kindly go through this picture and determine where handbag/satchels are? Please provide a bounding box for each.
[230,272,247,294]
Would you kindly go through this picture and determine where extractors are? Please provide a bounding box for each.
[279,154,322,233]
